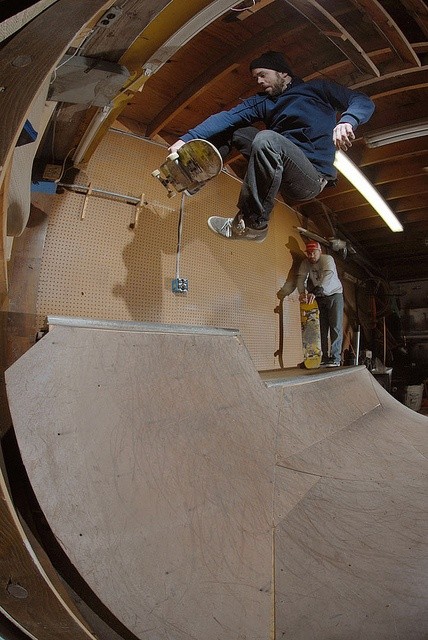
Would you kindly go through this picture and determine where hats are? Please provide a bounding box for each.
[250,50,292,76]
[304,241,321,252]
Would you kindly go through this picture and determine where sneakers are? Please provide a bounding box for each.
[326,361,340,367]
[320,361,325,368]
[207,211,268,244]
[167,153,201,196]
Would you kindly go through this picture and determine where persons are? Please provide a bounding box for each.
[298,240,343,367]
[167,51,376,243]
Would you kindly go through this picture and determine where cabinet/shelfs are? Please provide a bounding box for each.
[0,2,174,307]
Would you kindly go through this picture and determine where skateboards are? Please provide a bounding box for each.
[299,298,323,368]
[151,139,223,199]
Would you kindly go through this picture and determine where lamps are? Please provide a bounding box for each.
[334,150,406,233]
[362,118,428,149]
